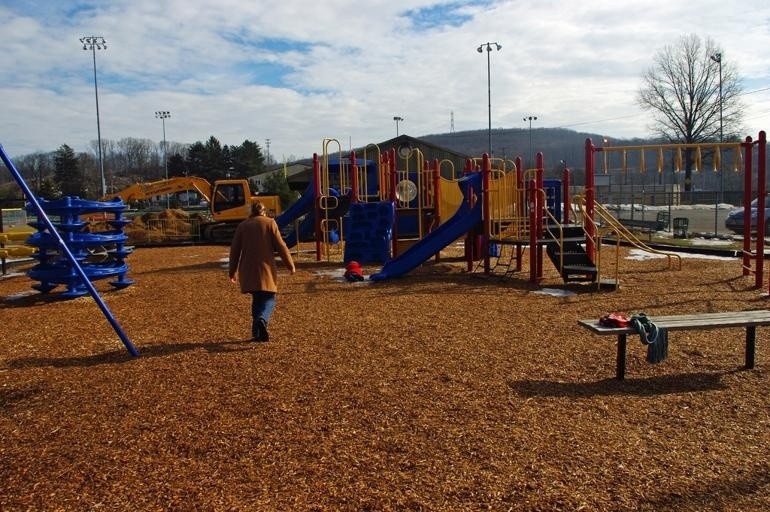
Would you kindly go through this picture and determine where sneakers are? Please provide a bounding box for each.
[252,318,269,342]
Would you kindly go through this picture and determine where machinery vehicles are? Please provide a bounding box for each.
[101,176,281,242]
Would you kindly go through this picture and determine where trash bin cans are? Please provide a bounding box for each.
[673,218,689,238]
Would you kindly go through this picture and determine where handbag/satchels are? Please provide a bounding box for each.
[600,314,632,327]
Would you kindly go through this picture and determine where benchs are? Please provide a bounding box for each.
[577,309,770,381]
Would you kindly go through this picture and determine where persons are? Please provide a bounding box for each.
[343,260,364,281]
[229,200,296,342]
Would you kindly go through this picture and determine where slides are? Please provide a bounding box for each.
[274,183,353,252]
[370,172,481,281]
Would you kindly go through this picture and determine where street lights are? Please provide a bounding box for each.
[523,114,538,170]
[155,110,171,210]
[475,40,503,157]
[713,53,726,205]
[79,34,108,197]
[393,116,404,137]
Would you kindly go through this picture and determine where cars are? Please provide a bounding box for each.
[725,194,770,235]
